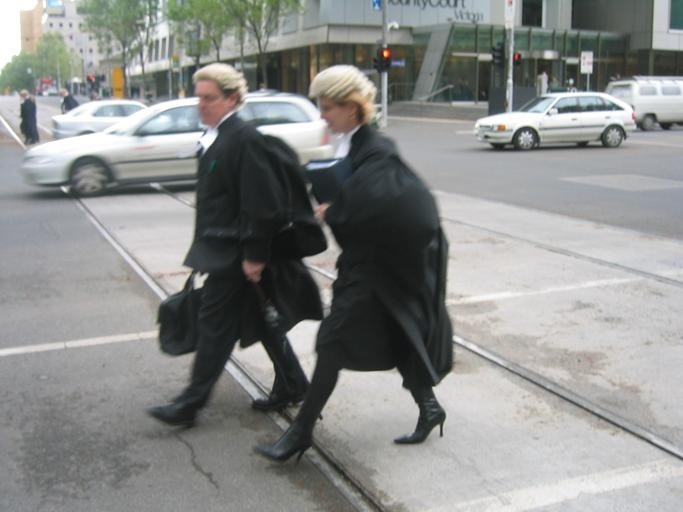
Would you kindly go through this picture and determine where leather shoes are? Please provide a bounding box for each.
[147,402,195,426]
[253,389,307,411]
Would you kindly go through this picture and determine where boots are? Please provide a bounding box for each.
[395,398,445,443]
[253,420,313,464]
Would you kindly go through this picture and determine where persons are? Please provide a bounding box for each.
[58,87,79,114]
[19,89,40,145]
[251,64,454,464]
[145,63,329,429]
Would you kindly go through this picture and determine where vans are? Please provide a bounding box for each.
[605,75,683,132]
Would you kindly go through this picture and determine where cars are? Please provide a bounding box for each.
[49,98,170,140]
[20,95,327,198]
[473,91,638,150]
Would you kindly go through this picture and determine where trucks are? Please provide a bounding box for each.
[37,79,56,94]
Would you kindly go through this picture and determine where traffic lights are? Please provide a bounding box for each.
[378,46,391,71]
[513,53,521,65]
[492,42,504,64]
[87,75,94,81]
[372,56,380,72]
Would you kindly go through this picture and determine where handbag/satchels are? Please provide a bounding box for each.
[159,269,205,355]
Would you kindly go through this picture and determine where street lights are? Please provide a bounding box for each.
[381,20,400,129]
